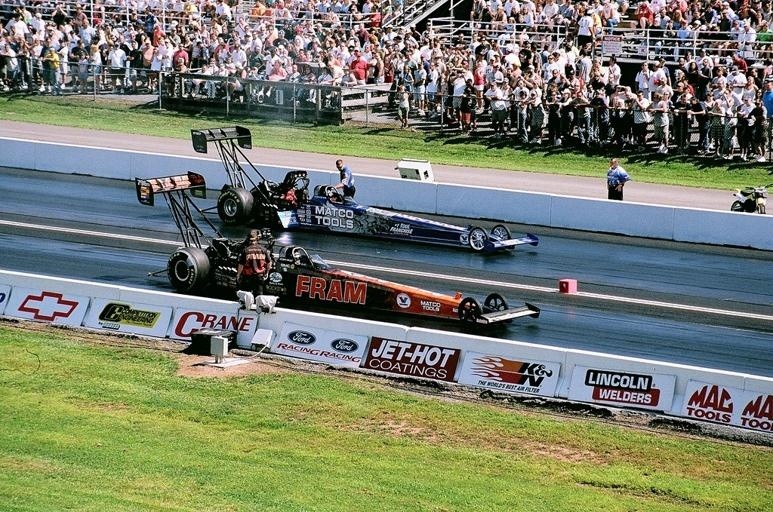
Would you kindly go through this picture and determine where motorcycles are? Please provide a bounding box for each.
[727,183,772,215]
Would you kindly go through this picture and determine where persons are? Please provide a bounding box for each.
[607,158,631,200]
[0,0,772,160]
[334,158,356,198]
[236,230,272,295]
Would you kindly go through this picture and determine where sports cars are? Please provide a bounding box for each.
[190,120,543,257]
[130,169,545,336]
